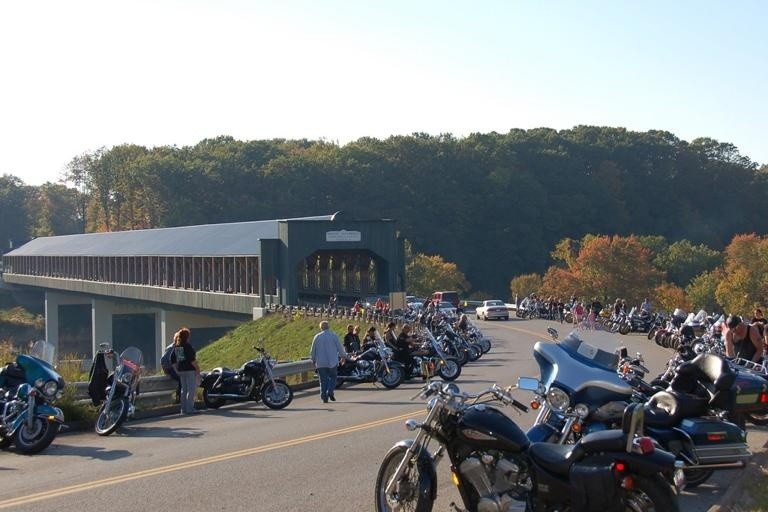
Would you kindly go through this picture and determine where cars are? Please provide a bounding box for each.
[475,299,510,322]
[354,295,382,311]
[406,290,461,314]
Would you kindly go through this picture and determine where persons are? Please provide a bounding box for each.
[169,326,201,416]
[762,323,768,347]
[546,293,652,334]
[158,330,181,400]
[723,315,764,365]
[748,308,767,334]
[330,292,468,369]
[309,318,350,402]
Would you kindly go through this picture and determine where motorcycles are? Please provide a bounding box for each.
[516,294,726,353]
[91,342,144,437]
[200,343,292,411]
[1,339,70,453]
[333,310,492,390]
